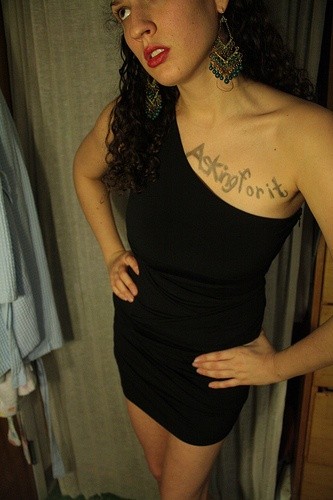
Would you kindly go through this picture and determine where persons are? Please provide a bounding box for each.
[73,0,333,500]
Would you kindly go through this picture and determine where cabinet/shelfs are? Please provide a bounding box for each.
[291,227,333,500]
